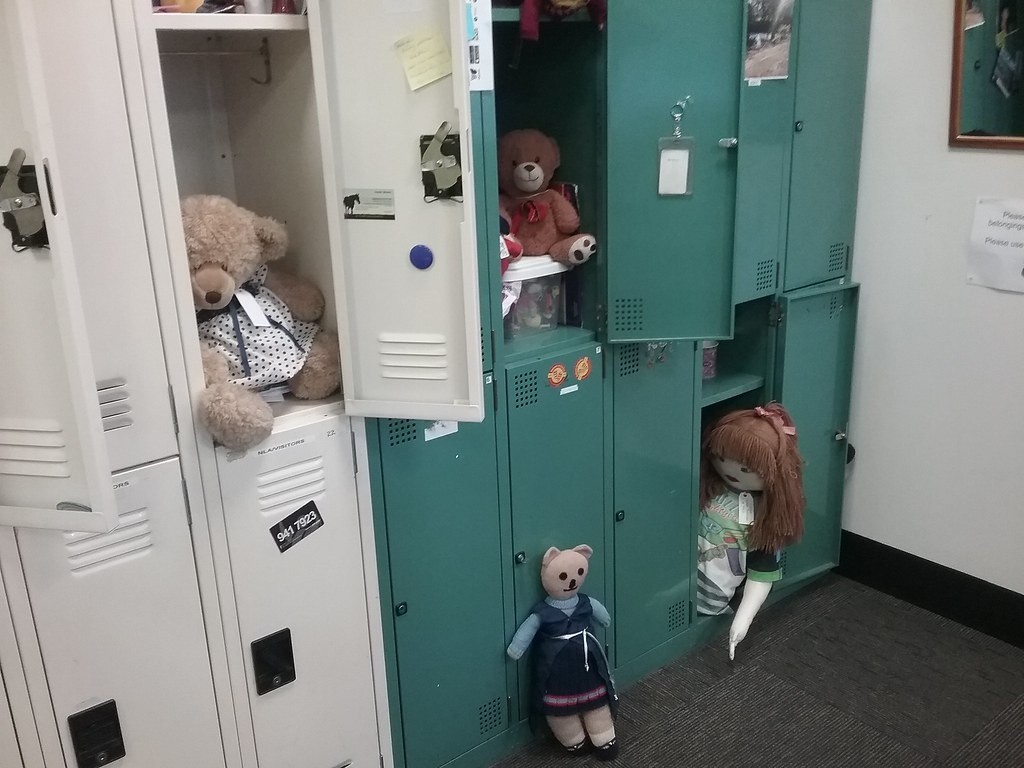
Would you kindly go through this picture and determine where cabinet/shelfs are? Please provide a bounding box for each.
[2,0,875,768]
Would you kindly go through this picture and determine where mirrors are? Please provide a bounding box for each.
[946,0,1024,152]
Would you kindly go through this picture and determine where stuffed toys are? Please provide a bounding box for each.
[499,126,598,275]
[507,542,622,760]
[177,194,341,451]
[697,399,808,661]
